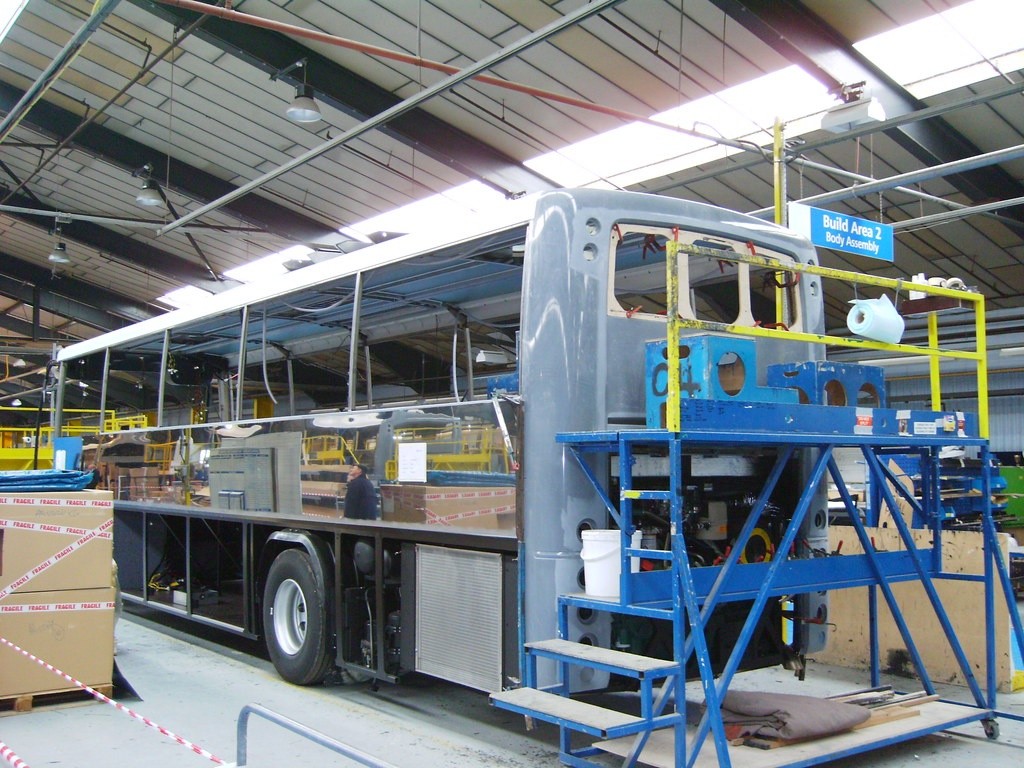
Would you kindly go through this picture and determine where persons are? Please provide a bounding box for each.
[343,464,377,521]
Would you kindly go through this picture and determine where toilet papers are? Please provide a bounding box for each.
[846,294,905,344]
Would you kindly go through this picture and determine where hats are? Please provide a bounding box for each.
[353,463,368,473]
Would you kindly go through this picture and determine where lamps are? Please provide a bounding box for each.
[12,377,24,406]
[270,58,324,124]
[821,83,887,135]
[14,357,25,367]
[132,159,162,206]
[476,328,508,364]
[48,226,71,264]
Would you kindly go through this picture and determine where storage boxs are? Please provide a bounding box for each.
[381,484,515,531]
[0,489,113,593]
[0,586,117,697]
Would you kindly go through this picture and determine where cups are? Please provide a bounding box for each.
[579,528,643,598]
